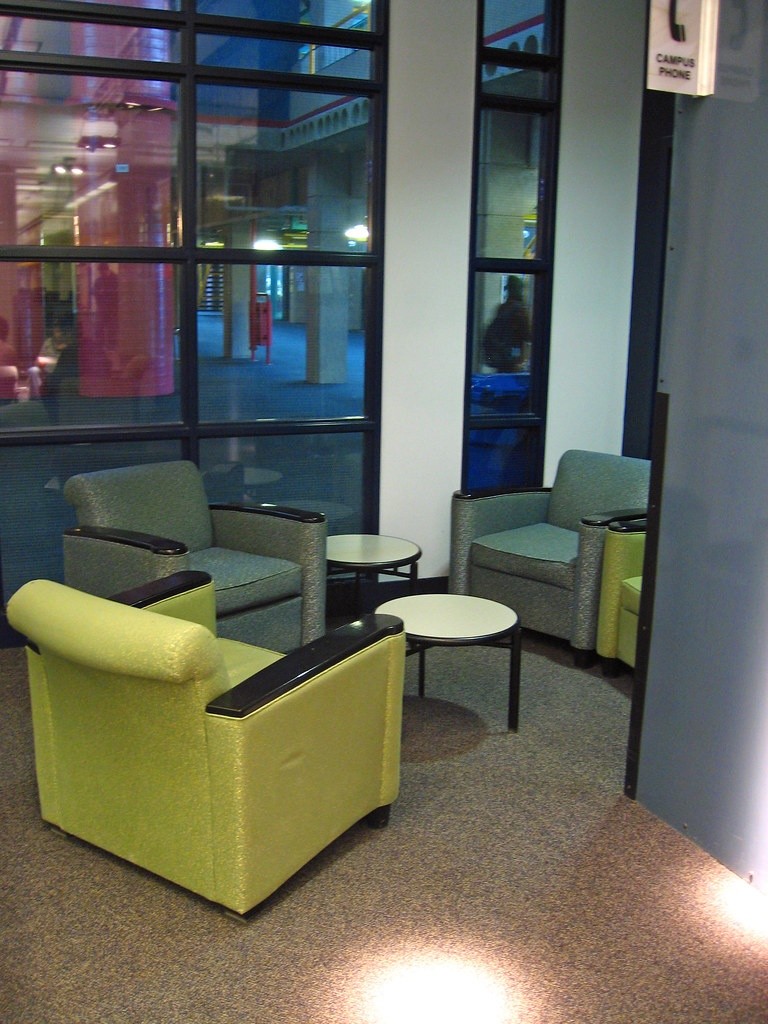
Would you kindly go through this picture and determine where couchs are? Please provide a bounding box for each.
[5,570,405,923]
[595,516,643,677]
[447,450,652,667]
[64,460,327,657]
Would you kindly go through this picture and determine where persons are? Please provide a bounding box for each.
[1,262,122,401]
[480,274,533,376]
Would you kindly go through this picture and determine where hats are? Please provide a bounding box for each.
[51,307,72,325]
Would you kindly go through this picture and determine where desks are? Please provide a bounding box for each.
[325,533,422,617]
[371,594,522,735]
